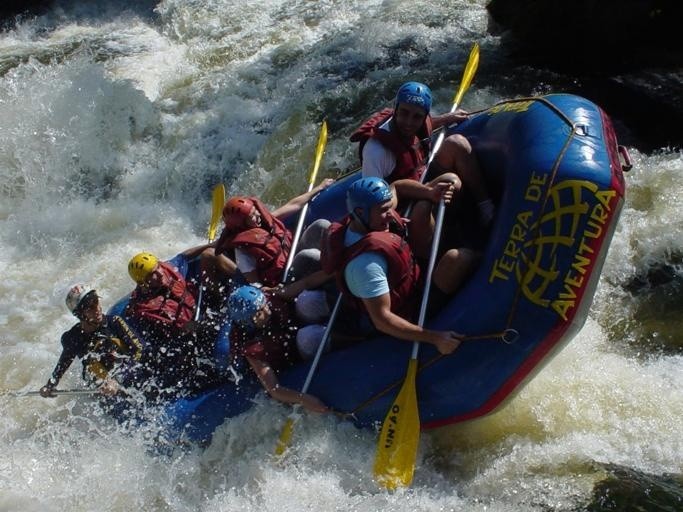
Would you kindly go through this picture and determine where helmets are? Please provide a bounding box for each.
[66,284,102,313]
[226,286,264,329]
[346,177,393,226]
[393,81,432,124]
[128,251,159,284]
[222,197,255,234]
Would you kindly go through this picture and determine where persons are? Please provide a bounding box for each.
[319,177,476,357]
[128,240,235,334]
[220,177,336,292]
[227,265,348,413]
[41,284,160,412]
[346,81,495,222]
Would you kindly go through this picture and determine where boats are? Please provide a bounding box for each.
[75,93,623,465]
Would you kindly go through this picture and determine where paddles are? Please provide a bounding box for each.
[194,185,224,320]
[373,192,445,487]
[402,44,480,217]
[274,293,344,456]
[283,121,327,280]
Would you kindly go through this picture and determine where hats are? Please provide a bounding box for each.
[79,291,99,309]
